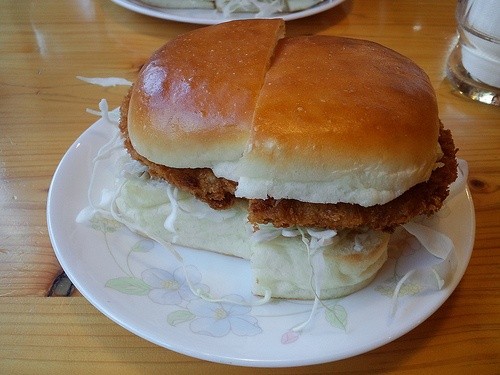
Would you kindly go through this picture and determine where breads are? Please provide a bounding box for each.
[128,18,440,210]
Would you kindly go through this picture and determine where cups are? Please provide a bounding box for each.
[443,0,499,109]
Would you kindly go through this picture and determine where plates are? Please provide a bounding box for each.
[113,0,343,31]
[47,100,477,369]
